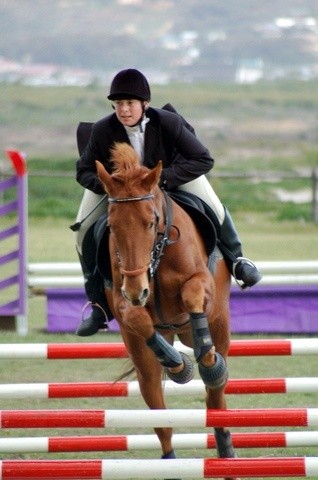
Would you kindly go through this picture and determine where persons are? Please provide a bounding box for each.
[74,69,262,336]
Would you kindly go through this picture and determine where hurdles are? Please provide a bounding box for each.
[0,150,318,480]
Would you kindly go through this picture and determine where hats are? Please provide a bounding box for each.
[107,68,150,100]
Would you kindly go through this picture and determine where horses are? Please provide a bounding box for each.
[92,140,242,480]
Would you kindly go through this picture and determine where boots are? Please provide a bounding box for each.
[76,245,114,336]
[217,205,263,286]
